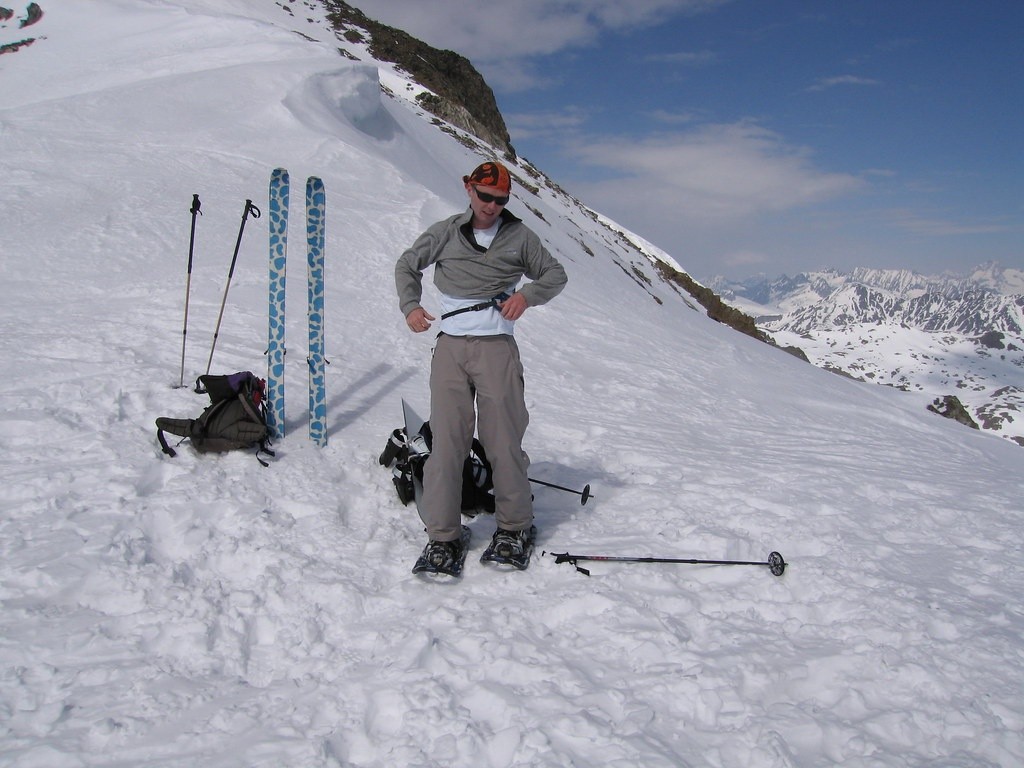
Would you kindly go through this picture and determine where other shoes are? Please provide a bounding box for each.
[426,540,460,567]
[492,528,528,558]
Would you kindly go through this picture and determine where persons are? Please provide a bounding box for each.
[396,160,568,583]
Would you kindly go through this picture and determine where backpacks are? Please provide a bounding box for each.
[156,372,276,466]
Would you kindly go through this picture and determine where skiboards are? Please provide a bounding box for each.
[267,167,290,439]
[305,176,328,447]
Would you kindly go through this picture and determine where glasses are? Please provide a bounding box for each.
[471,185,509,206]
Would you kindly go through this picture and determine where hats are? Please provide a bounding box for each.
[462,162,511,193]
